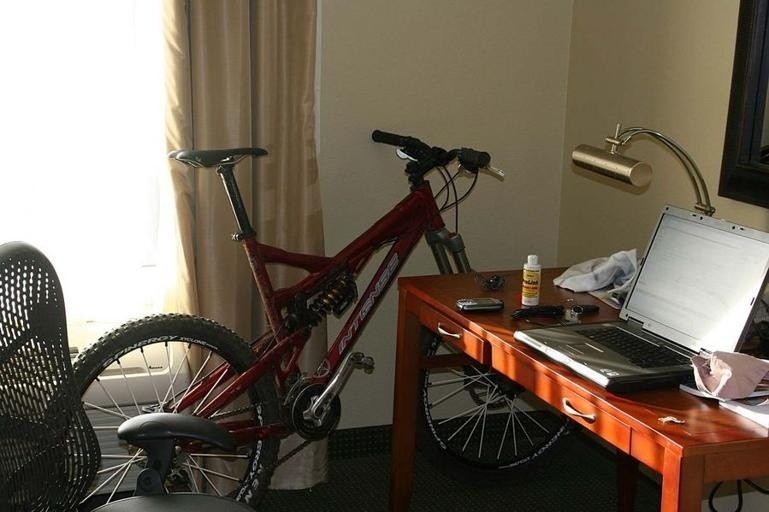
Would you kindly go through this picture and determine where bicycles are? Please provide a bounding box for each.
[42,129,582,512]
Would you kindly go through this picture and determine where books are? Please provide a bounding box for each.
[679,379,769,429]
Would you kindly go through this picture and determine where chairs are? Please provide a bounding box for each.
[1,240,259,512]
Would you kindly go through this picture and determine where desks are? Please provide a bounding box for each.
[390,263,769,511]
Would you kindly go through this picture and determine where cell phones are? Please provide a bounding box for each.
[456,297,505,312]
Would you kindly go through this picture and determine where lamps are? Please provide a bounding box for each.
[567,122,718,217]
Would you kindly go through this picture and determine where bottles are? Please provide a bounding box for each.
[522,254,542,306]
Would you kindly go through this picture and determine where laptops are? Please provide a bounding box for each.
[513,205,768,392]
